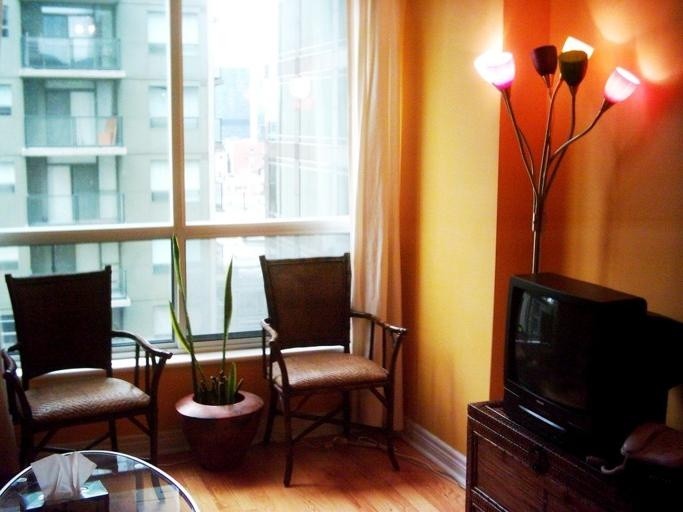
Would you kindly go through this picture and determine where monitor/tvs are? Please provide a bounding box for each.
[502,271,683,472]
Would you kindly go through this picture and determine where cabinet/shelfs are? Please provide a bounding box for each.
[465,403,682,511]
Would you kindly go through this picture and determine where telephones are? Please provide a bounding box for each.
[620,420,683,466]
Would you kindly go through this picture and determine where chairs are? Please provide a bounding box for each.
[1,267,172,511]
[258,251,405,486]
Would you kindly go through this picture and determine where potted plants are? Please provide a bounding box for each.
[165,235,263,468]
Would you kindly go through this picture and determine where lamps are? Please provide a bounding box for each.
[472,35,641,273]
